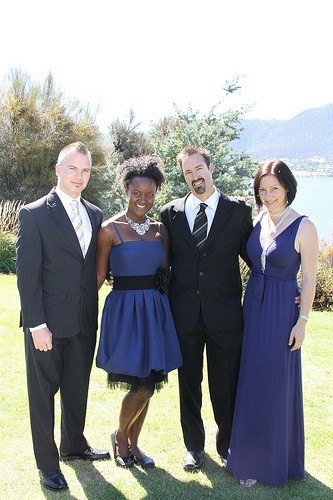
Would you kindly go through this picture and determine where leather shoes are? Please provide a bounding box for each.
[59,444,110,461]
[39,466,69,492]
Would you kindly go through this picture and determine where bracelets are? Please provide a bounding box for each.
[298,314,310,322]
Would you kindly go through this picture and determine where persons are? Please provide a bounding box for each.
[109,146,301,471]
[95,154,185,470]
[224,160,320,487]
[16,141,111,491]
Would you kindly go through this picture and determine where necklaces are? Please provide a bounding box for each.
[125,207,150,236]
[266,208,290,234]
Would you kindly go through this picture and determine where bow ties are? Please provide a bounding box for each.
[69,198,86,257]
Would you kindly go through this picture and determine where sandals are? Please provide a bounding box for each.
[240,479,258,487]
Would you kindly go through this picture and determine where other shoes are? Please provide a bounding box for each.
[182,447,205,470]
[219,456,228,465]
[110,430,136,469]
[129,445,156,469]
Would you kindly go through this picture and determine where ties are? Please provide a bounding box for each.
[192,202,208,255]
